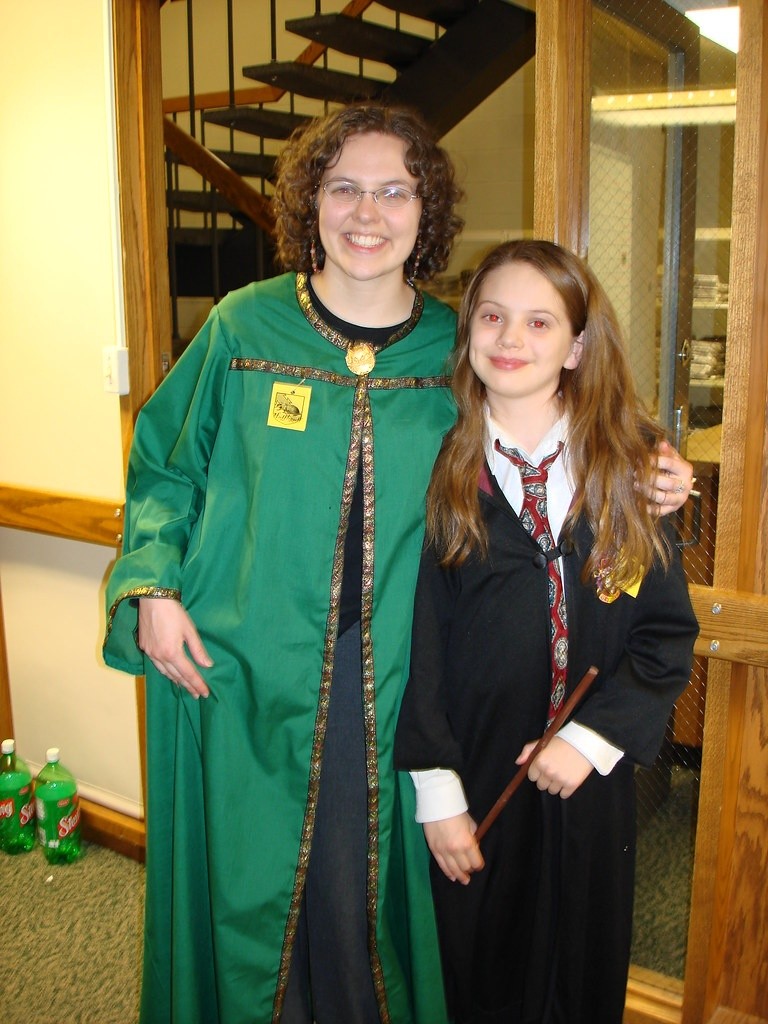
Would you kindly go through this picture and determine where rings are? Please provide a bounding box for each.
[672,478,684,496]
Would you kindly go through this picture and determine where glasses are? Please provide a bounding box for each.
[324,180,419,209]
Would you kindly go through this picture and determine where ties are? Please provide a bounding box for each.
[494,437,569,730]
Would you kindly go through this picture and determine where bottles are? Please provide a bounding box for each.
[0,739,39,854]
[34,747,82,864]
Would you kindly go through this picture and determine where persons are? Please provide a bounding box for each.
[105,105,689,1021]
[389,240,695,1021]
